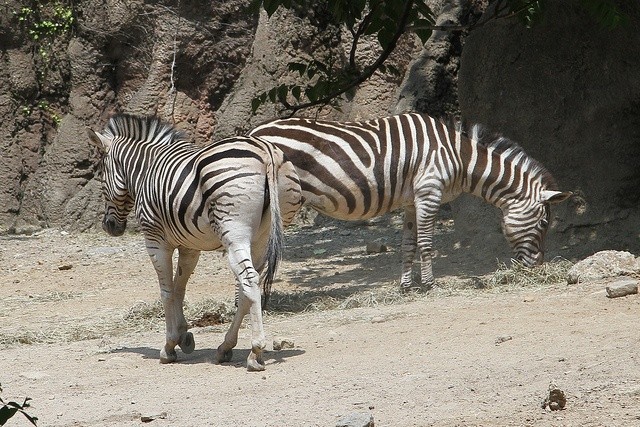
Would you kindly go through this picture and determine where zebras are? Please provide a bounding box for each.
[84,111,303,373]
[234,110,575,311]
